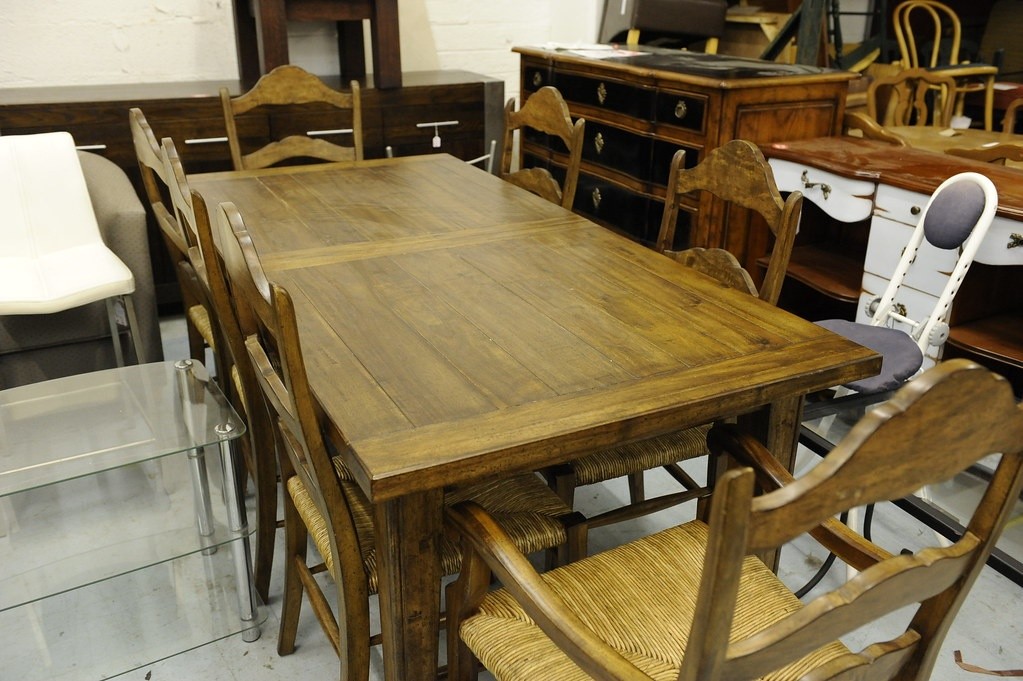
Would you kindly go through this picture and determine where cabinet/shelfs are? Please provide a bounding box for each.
[511,44,862,283]
[754,131,1023,392]
[0,68,504,316]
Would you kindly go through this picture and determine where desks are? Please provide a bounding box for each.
[183,153,885,681]
[0,358,269,681]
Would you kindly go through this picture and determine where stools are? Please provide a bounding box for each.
[231,0,402,91]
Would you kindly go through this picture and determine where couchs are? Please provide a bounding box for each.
[0,148,164,391]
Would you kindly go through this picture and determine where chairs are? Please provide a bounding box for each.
[626,0,727,54]
[865,67,956,129]
[0,132,145,368]
[943,141,1023,166]
[842,111,911,147]
[893,0,998,133]
[128,64,1023,681]
